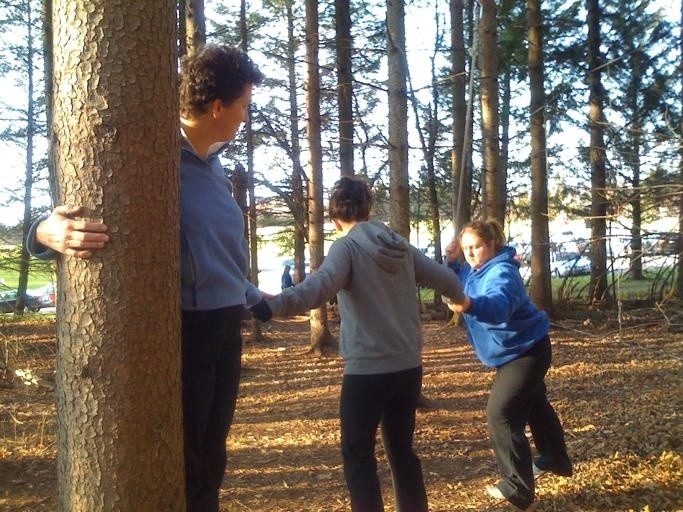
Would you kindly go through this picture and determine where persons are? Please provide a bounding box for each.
[443,220,573,510]
[281,265,293,290]
[249,176,464,512]
[25,43,261,511]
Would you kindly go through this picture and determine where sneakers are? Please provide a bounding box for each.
[487,482,522,502]
[532,459,561,474]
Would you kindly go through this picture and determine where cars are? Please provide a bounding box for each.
[282,253,319,289]
[33,283,55,309]
[0,291,52,314]
[511,221,680,286]
[417,245,448,265]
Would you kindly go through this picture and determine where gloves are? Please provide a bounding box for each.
[248,301,272,325]
